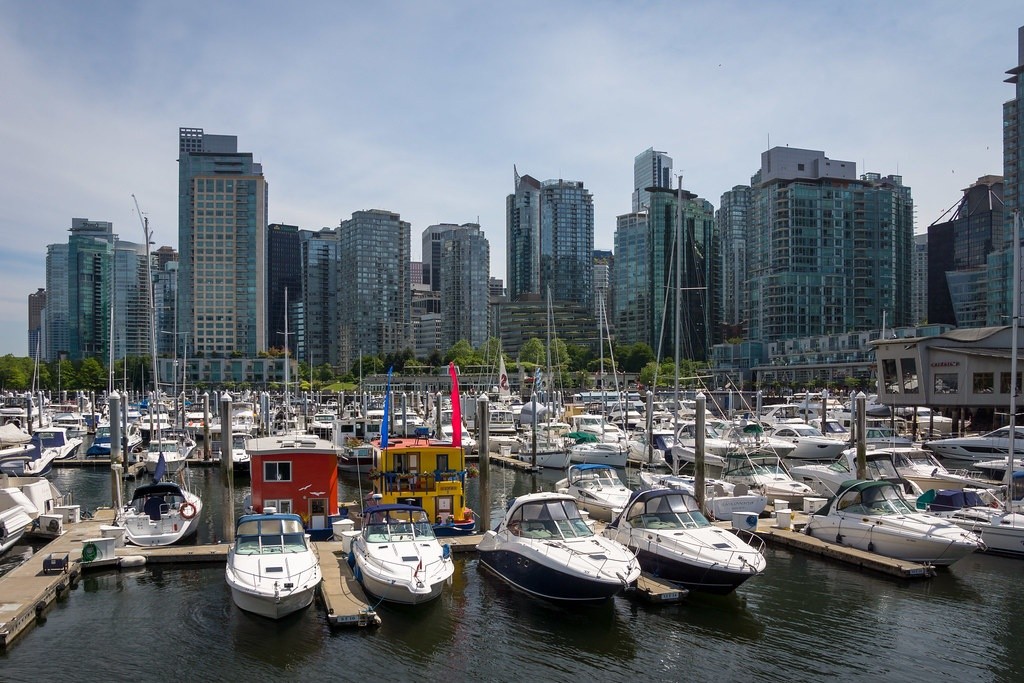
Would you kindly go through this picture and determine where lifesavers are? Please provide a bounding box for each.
[180,503,196,519]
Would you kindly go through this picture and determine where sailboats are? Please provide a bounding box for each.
[101,215,203,548]
[487,282,972,467]
[0,305,471,476]
[363,362,478,536]
[719,388,821,502]
[940,206,1024,553]
[639,169,769,520]
[243,285,350,540]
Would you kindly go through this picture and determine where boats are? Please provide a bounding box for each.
[922,422,1024,461]
[597,487,767,596]
[787,432,1004,495]
[0,469,63,557]
[554,461,645,520]
[800,476,985,571]
[341,493,456,608]
[474,492,642,606]
[224,506,324,621]
[785,442,1006,498]
